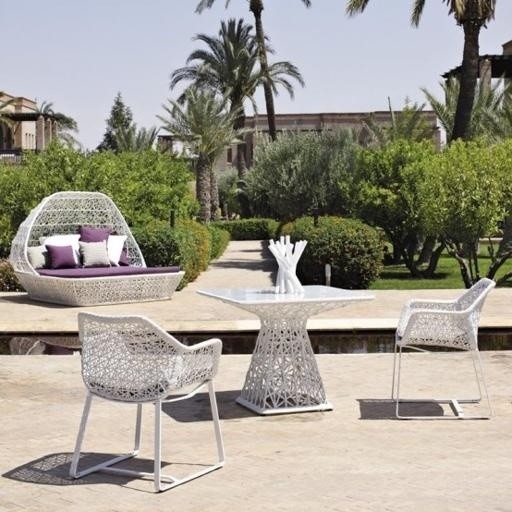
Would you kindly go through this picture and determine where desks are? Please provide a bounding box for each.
[197,284,374,416]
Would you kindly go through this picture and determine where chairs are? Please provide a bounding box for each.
[70,311,225,492]
[391,275,497,418]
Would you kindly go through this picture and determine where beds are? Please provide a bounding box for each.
[10,192,185,306]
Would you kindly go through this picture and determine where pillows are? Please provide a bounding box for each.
[28,226,130,269]
